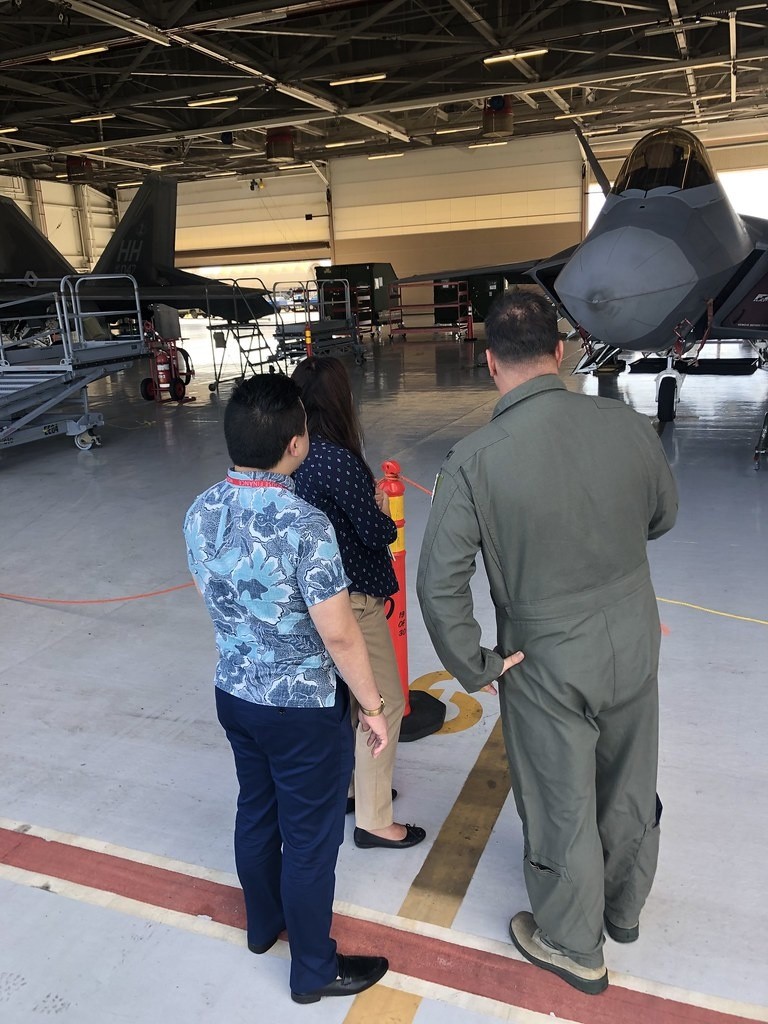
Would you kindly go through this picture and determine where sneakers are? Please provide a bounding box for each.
[509,911,609,995]
[604,911,639,943]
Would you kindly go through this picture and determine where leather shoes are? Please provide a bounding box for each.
[291,952,389,1004]
[354,823,426,849]
[346,789,397,814]
[248,927,288,954]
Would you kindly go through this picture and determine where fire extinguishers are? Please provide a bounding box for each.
[147,346,172,388]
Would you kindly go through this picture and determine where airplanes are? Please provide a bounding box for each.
[1,174,282,336]
[388,121,768,423]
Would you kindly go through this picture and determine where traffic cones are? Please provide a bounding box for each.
[378,461,446,743]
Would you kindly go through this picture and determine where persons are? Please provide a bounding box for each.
[287,355,426,850]
[415,288,681,995]
[183,372,390,1005]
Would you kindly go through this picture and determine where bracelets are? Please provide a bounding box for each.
[359,694,386,717]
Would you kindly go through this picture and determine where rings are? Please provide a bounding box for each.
[382,496,384,500]
